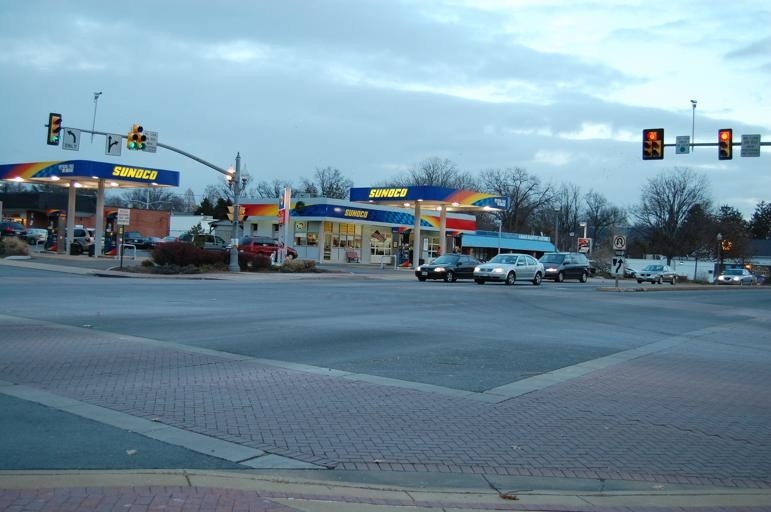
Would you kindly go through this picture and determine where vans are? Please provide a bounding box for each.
[535,249,595,285]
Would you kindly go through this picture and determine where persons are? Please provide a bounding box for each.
[44,221,54,250]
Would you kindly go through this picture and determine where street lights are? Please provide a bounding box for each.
[579,221,586,238]
[552,206,560,246]
[493,218,501,250]
[225,151,249,273]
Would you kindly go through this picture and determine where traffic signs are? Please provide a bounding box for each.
[142,130,159,154]
[740,134,761,158]
[103,133,123,156]
[675,135,690,154]
[61,126,81,152]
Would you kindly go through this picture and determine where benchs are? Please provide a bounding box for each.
[346,251,361,263]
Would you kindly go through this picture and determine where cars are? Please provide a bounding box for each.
[716,268,758,288]
[634,262,679,287]
[471,251,547,287]
[413,253,484,283]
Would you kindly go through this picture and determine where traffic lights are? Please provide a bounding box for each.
[646,131,659,141]
[717,128,733,161]
[132,123,144,134]
[651,140,663,158]
[127,131,138,151]
[45,112,62,145]
[137,133,147,150]
[643,140,653,158]
